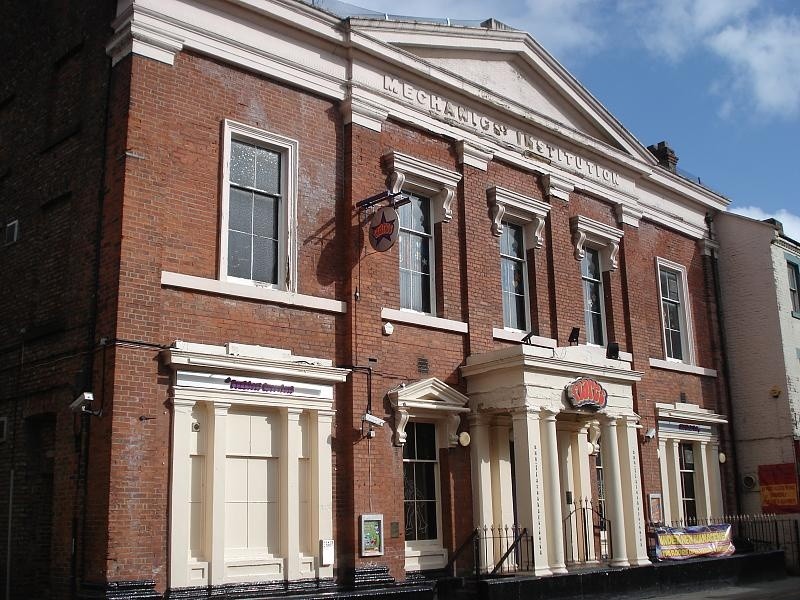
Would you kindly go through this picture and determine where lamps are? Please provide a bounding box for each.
[606,342,621,359]
[521,331,533,345]
[568,327,580,346]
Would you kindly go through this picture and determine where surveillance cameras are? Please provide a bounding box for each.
[645,428,655,439]
[69,392,94,412]
[362,413,386,427]
